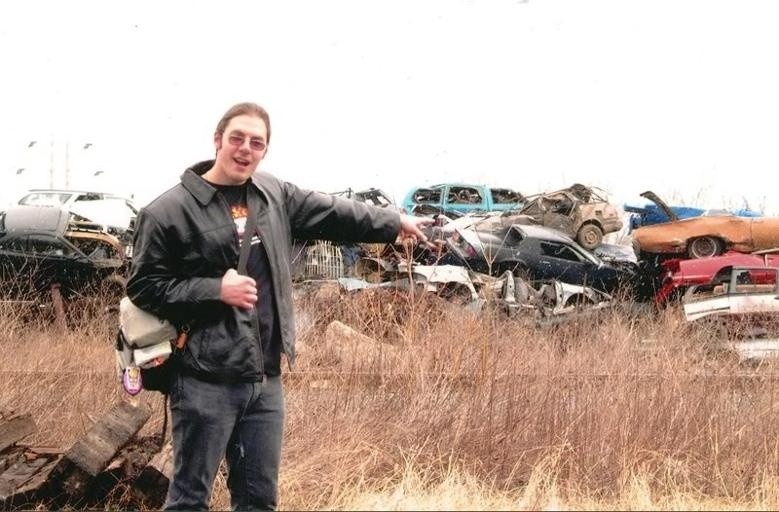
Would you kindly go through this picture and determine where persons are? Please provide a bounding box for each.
[116,105,437,512]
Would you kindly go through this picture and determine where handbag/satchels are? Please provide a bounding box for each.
[115,298,177,394]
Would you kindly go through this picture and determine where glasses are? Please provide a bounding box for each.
[230,136,266,152]
